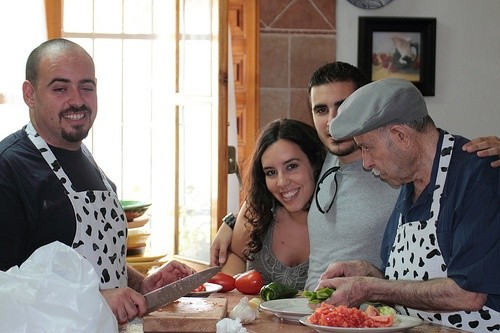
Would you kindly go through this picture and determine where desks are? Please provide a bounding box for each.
[122,288,471,333]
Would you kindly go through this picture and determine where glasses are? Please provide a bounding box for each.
[316,166,340,215]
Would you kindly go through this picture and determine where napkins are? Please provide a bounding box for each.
[215,318,247,333]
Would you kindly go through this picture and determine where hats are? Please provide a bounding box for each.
[328,79,428,140]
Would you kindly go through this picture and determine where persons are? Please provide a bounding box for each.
[307,77,500,333]
[219,118,328,293]
[0,39,197,325]
[211,61,500,293]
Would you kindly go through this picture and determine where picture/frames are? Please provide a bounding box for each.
[358,15,437,96]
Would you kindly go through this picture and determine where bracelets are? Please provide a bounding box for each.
[222,213,237,231]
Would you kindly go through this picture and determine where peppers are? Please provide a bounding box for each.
[260,281,298,301]
[304,287,334,304]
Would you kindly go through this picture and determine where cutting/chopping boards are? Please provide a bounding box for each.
[143,297,228,333]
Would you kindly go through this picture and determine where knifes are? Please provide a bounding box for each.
[132,266,223,314]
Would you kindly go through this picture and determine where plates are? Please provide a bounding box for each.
[260,298,315,322]
[299,314,423,333]
[119,201,168,274]
[188,282,223,297]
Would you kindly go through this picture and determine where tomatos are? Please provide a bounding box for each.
[208,272,236,293]
[235,270,265,294]
[192,285,206,292]
[305,305,394,328]
